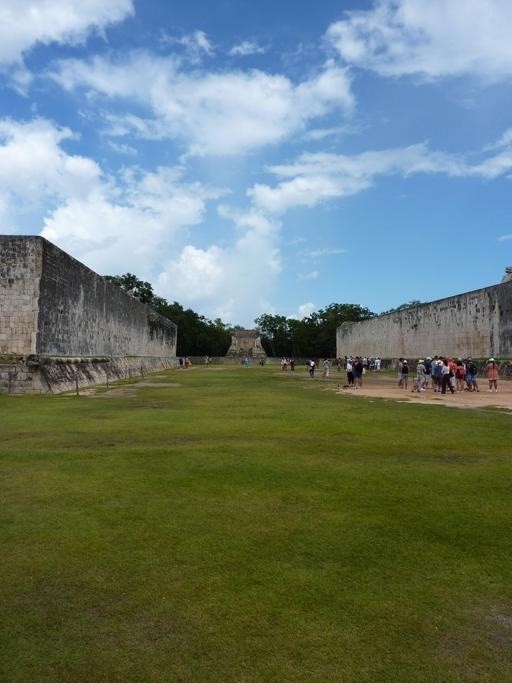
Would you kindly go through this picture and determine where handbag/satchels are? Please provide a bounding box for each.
[450,368,455,378]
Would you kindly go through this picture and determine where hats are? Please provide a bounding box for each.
[402,360,408,363]
[488,357,494,361]
[414,359,425,364]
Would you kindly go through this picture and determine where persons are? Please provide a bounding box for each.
[240,350,265,368]
[485,356,499,391]
[322,357,330,377]
[178,354,192,368]
[336,354,481,394]
[309,359,316,377]
[201,354,213,367]
[280,355,295,370]
